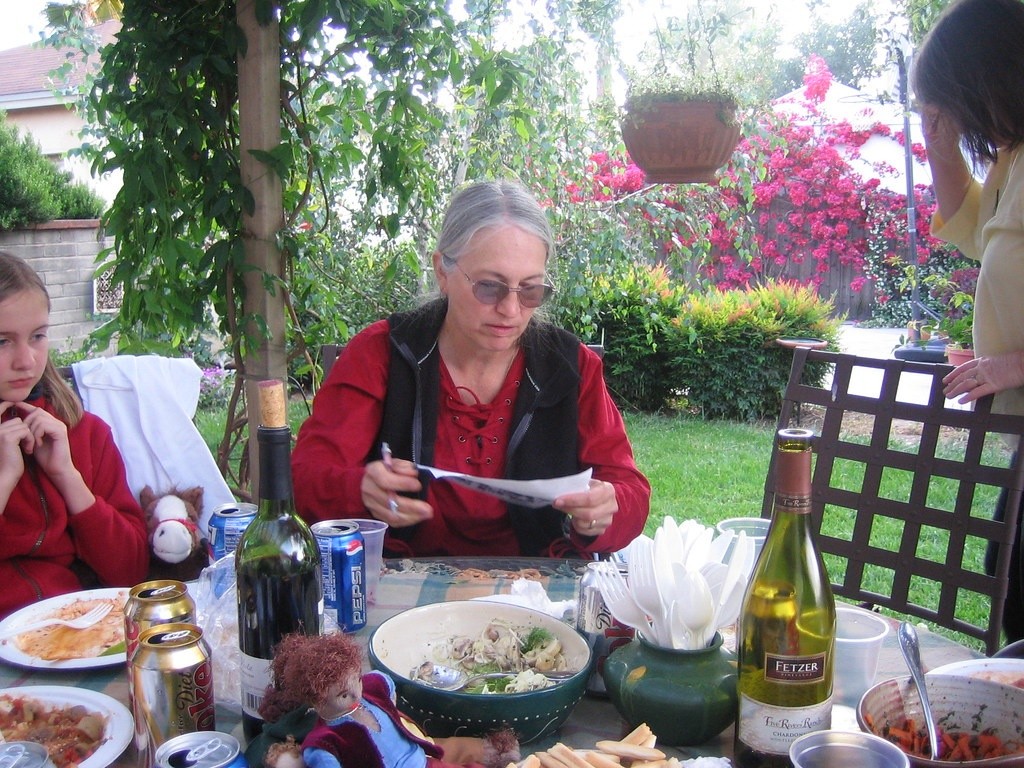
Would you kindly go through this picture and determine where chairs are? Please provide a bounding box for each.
[763,338,1024,655]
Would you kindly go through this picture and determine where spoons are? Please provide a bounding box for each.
[677,571,714,650]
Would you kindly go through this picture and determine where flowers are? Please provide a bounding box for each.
[885,255,980,356]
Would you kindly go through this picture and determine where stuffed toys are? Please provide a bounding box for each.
[140,484,211,582]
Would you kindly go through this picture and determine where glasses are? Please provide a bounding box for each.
[443,254,559,308]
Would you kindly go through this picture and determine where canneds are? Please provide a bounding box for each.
[208,502,259,601]
[123,579,250,768]
[0,741,60,768]
[576,561,640,699]
[308,519,368,635]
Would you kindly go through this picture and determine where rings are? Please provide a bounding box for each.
[972,376,980,386]
[590,519,596,529]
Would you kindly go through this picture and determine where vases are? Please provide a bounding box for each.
[908,325,930,340]
[945,344,975,366]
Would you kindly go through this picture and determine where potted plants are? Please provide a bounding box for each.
[617,60,780,184]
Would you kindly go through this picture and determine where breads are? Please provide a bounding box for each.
[505,723,682,768]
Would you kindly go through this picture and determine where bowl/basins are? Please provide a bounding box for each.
[369,600,594,746]
[857,675,1024,768]
[923,659,1024,688]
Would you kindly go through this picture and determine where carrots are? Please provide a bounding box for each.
[864,714,1024,761]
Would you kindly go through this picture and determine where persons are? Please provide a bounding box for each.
[258,631,520,768]
[290,180,651,558]
[911,0,1024,658]
[0,252,148,621]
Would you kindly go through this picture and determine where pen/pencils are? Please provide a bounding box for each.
[381,442,398,513]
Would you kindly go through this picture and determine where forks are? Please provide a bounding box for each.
[0,603,114,639]
[594,542,668,648]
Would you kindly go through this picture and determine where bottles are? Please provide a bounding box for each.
[234,380,323,745]
[734,428,836,768]
[604,630,738,746]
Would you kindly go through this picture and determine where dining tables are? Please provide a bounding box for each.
[0,556,987,768]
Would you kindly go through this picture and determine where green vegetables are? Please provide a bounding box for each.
[455,625,554,694]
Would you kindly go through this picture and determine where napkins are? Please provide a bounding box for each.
[473,578,575,621]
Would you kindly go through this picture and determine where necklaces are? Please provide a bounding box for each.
[320,705,359,722]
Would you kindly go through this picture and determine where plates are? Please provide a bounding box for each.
[0,588,131,668]
[0,686,134,768]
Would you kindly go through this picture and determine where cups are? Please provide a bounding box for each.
[342,519,389,611]
[790,729,911,768]
[715,518,770,577]
[796,609,890,729]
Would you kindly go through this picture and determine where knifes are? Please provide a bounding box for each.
[653,515,755,647]
